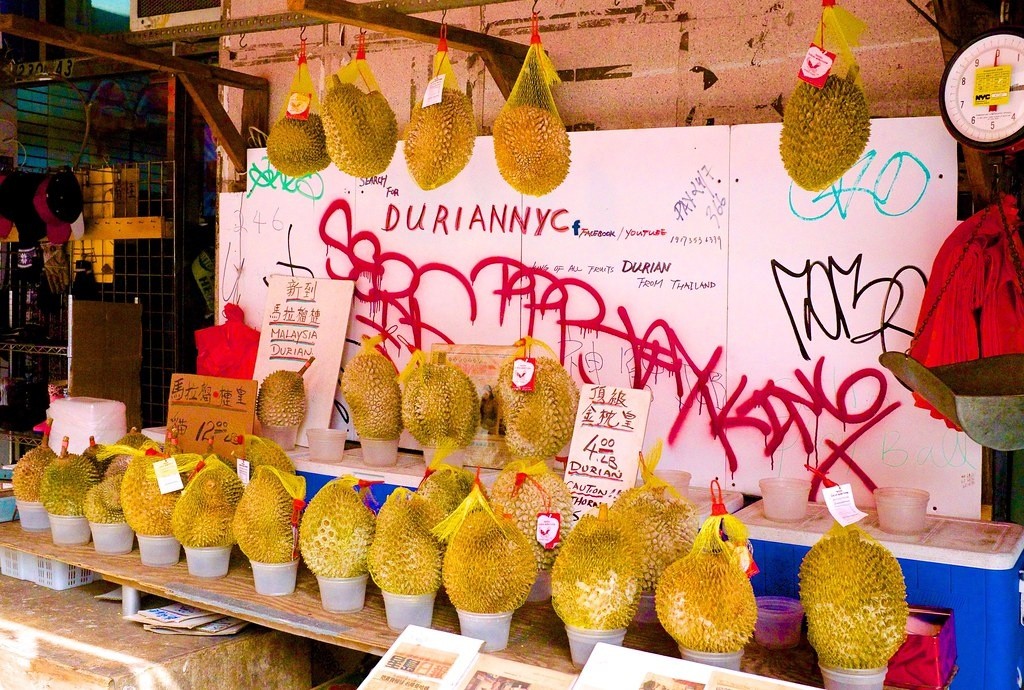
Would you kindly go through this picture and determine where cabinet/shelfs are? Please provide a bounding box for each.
[0,296,142,448]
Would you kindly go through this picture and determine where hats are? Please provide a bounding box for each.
[0,165,85,244]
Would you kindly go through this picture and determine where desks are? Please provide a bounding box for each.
[0,516,959,690]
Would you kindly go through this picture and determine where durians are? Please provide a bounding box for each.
[320,73,399,178]
[778,65,873,192]
[254,356,316,427]
[400,350,481,448]
[266,113,332,178]
[403,86,478,191]
[797,530,909,670]
[492,105,572,196]
[496,354,582,461]
[12,416,757,654]
[341,353,405,439]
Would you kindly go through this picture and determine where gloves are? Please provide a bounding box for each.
[72,260,97,301]
[41,242,69,293]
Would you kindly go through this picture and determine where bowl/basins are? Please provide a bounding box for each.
[632,592,660,624]
[526,570,551,604]
[564,625,627,670]
[260,423,556,472]
[456,608,514,653]
[818,660,888,690]
[654,469,692,499]
[873,486,930,536]
[755,595,804,650]
[759,478,812,523]
[316,575,368,614]
[678,644,744,671]
[380,590,436,632]
[14,498,300,597]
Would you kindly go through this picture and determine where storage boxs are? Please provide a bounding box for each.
[0,546,94,593]
[292,447,501,516]
[883,605,958,690]
[732,500,1024,690]
[141,427,313,460]
[689,486,745,532]
